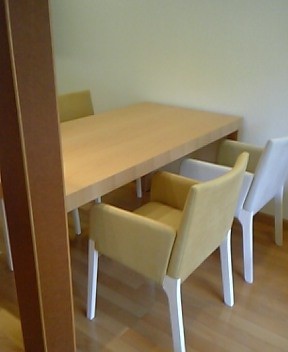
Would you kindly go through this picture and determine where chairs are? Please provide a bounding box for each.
[57,88,93,124]
[178,138,288,283]
[86,151,250,352]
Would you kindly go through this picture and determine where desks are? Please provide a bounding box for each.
[58,99,244,235]
